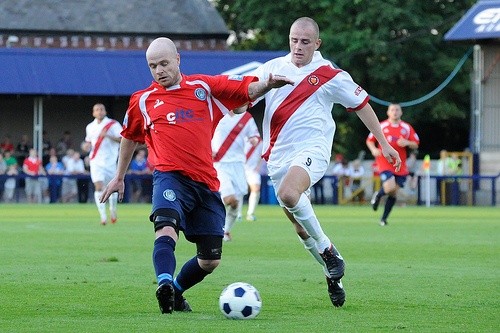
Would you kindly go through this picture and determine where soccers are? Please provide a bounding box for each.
[219,281,262,320]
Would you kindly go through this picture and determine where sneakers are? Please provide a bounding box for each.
[326,275,346,307]
[156,281,175,314]
[370,191,380,210]
[173,295,193,312]
[319,241,345,283]
[380,218,386,226]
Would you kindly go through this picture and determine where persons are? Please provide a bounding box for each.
[0,130,94,204]
[236,114,263,223]
[80,103,124,225]
[405,150,419,191]
[333,159,365,201]
[437,151,463,175]
[98,37,295,314]
[241,16,402,307]
[365,102,420,226]
[210,104,260,241]
[125,149,152,202]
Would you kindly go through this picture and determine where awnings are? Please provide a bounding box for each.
[0,46,291,166]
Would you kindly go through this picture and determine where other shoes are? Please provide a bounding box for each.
[223,233,231,241]
